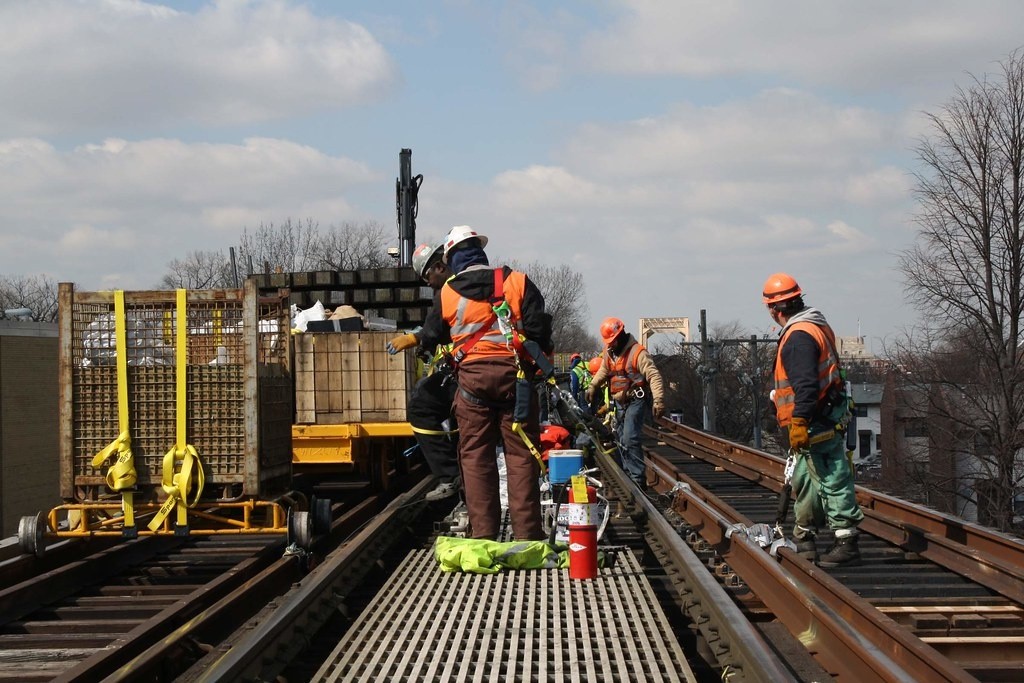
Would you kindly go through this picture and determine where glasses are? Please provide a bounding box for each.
[422,260,441,282]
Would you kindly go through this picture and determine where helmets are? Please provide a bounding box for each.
[571,354,579,362]
[411,241,443,277]
[589,358,603,374]
[600,317,624,343]
[762,273,803,304]
[442,225,488,265]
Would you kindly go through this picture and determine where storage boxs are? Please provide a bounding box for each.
[550,482,570,529]
[58,279,296,500]
[180,334,293,367]
[295,332,418,424]
[548,449,584,484]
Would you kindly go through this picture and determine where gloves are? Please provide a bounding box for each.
[652,397,666,419]
[385,332,418,355]
[585,384,597,403]
[789,418,809,452]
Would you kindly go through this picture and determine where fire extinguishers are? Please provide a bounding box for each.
[567,466,603,580]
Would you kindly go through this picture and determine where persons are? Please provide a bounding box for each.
[422,224,555,542]
[584,317,664,499]
[384,240,470,532]
[588,357,617,442]
[530,347,555,427]
[761,272,866,569]
[569,352,593,420]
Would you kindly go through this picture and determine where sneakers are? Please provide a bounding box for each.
[792,538,815,559]
[426,475,462,500]
[818,539,861,568]
[635,481,648,491]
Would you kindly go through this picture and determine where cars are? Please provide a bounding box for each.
[854,450,882,475]
